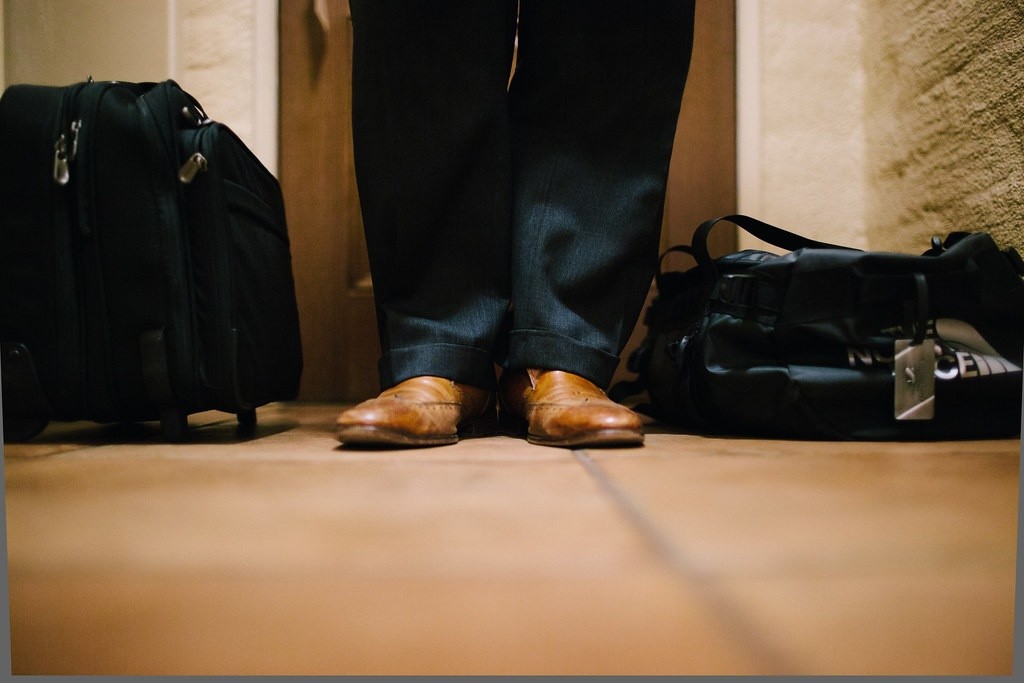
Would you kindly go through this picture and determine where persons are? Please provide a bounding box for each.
[335,0,695,448]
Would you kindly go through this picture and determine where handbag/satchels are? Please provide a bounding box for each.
[0,78,304,440]
[607,214,1023,440]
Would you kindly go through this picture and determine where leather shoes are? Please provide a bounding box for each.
[336,376,498,446]
[499,368,645,447]
